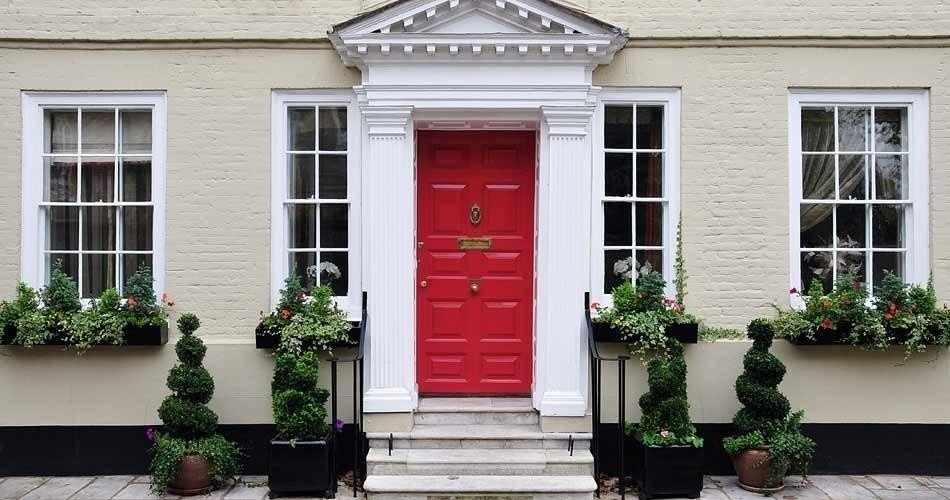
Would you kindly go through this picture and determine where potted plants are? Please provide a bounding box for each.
[882,322,944,346]
[722,317,817,493]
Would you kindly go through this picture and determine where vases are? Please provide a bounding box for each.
[785,322,852,347]
[167,453,215,496]
[627,427,703,500]
[267,432,334,499]
[590,316,699,344]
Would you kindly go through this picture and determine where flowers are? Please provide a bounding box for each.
[590,292,681,315]
[307,261,341,286]
[267,350,345,441]
[255,269,360,358]
[624,339,705,448]
[145,313,251,497]
[787,275,920,327]
[0,258,175,359]
